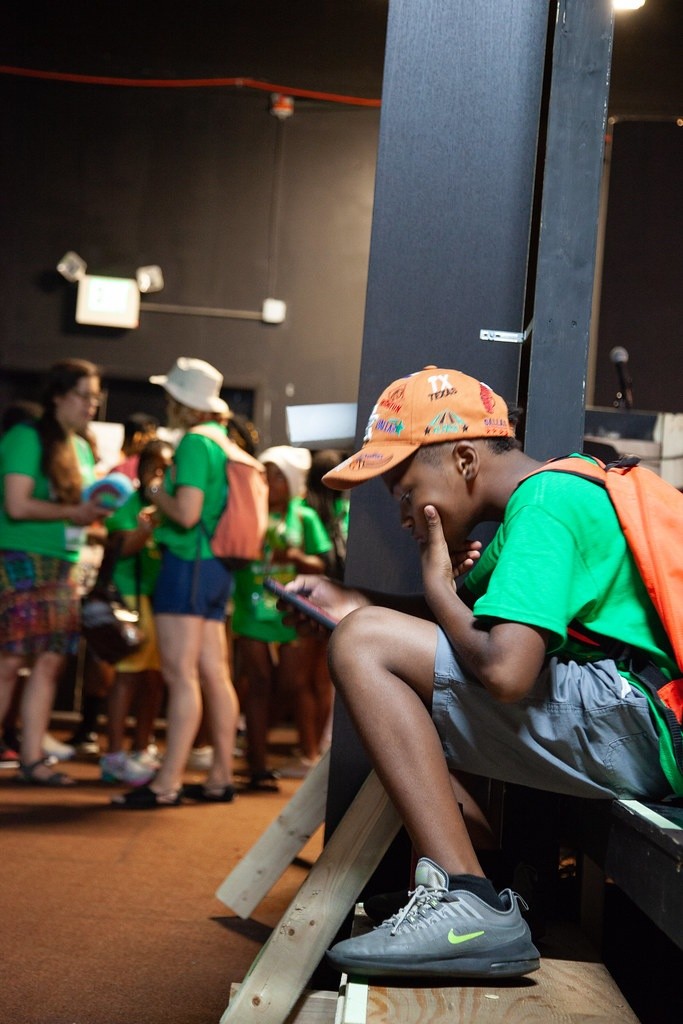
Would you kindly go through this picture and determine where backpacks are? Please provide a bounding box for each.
[508,453,683,770]
[171,424,270,573]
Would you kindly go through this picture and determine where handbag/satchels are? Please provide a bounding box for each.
[78,529,149,666]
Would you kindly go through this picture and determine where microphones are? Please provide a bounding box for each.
[610,346,633,408]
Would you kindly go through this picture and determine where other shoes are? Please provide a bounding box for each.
[0,732,99,768]
[101,745,322,794]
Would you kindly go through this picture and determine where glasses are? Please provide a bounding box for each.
[73,390,107,405]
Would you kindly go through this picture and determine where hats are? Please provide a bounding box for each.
[149,357,229,413]
[322,366,516,491]
[259,445,311,498]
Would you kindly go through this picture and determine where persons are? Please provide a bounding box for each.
[274,364,683,976]
[0,355,349,809]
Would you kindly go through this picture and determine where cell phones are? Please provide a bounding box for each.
[262,578,339,632]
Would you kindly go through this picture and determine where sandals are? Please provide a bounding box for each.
[15,760,75,788]
[109,782,232,810]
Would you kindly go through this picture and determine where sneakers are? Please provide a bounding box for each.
[326,857,538,978]
